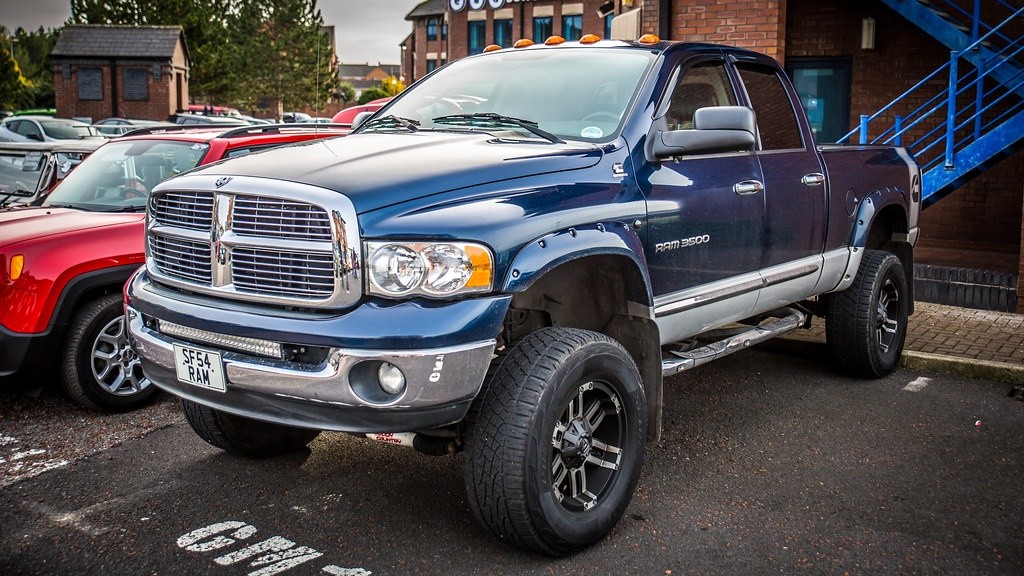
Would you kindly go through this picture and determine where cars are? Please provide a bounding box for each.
[0,94,487,419]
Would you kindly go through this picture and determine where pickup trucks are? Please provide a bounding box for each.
[123,31,925,562]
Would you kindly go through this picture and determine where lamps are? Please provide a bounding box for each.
[861,18,875,51]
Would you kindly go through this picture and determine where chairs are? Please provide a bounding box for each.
[141,151,176,190]
[669,83,720,132]
